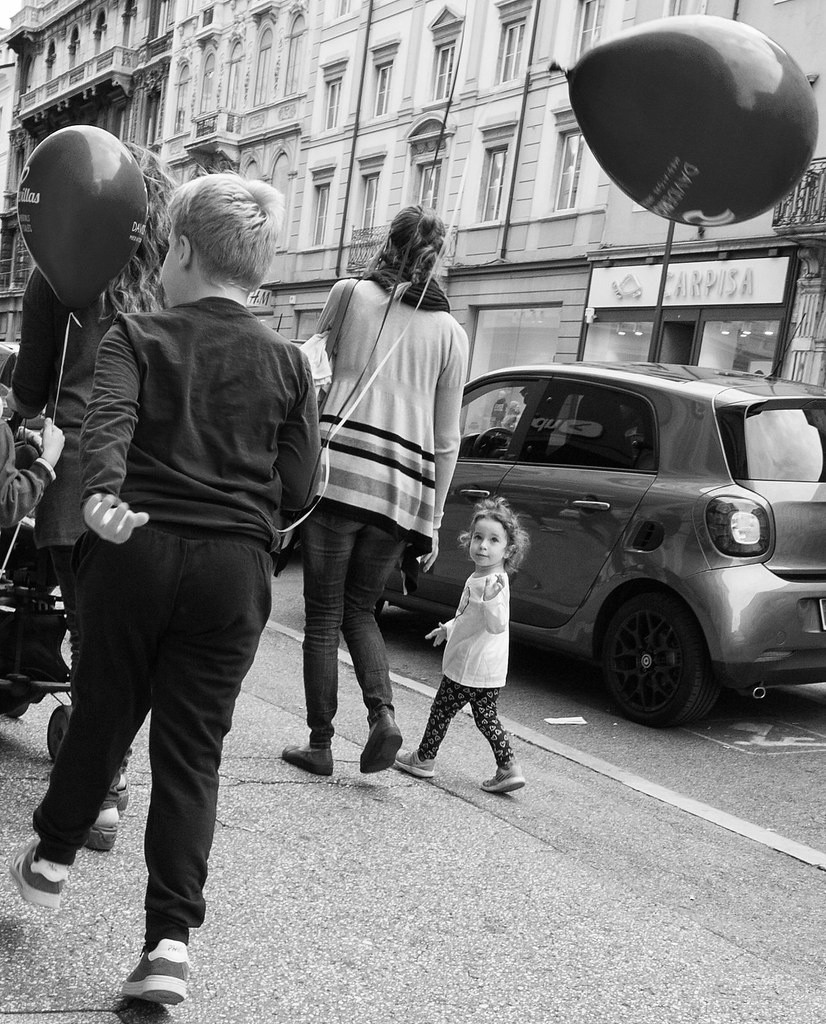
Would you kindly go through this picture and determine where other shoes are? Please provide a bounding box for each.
[281,744,335,777]
[360,714,403,774]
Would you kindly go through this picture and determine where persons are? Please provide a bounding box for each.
[398,498,532,796]
[0,140,317,1006]
[281,205,469,778]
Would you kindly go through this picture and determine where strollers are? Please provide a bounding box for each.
[0,419,78,761]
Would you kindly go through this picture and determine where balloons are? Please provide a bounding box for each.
[18,122,149,325]
[549,13,821,227]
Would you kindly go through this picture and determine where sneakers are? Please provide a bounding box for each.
[8,838,72,911]
[479,761,527,796]
[389,748,436,779]
[119,937,191,1006]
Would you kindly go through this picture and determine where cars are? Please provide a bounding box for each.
[374,362,826,726]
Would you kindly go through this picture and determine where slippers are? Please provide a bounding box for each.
[115,789,129,810]
[82,824,118,852]
